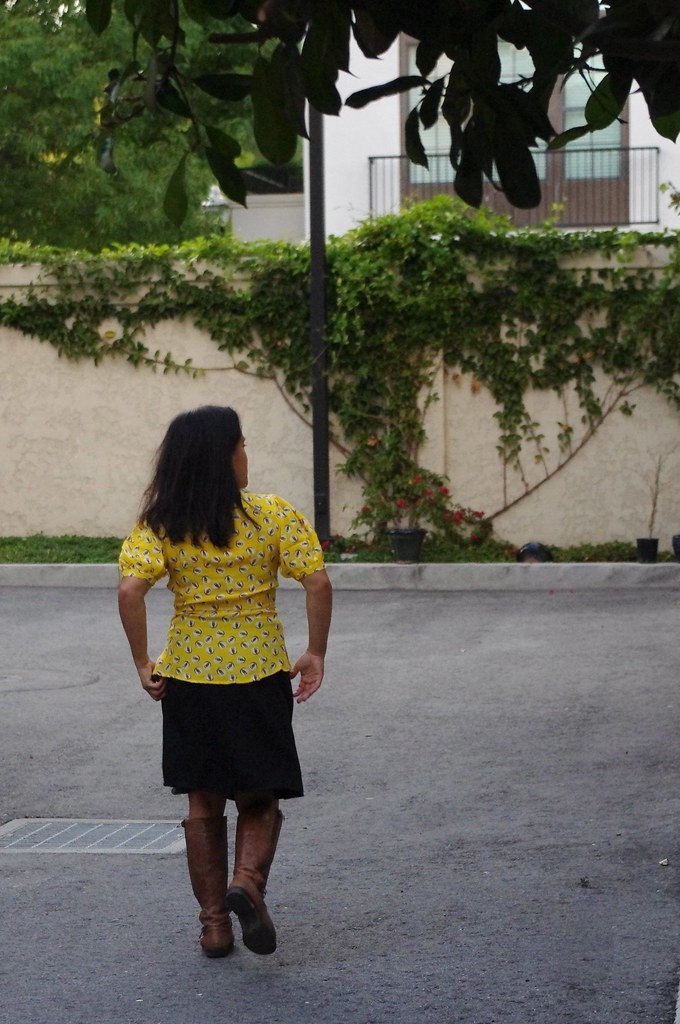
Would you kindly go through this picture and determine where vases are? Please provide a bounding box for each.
[389,528,428,561]
[672,535,680,561]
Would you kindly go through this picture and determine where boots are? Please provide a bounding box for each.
[225,808,284,953]
[180,815,233,956]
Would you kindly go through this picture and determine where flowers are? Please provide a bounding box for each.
[348,465,493,543]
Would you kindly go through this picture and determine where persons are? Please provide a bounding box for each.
[116,403,334,956]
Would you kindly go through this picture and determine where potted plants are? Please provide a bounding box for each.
[634,442,680,563]
